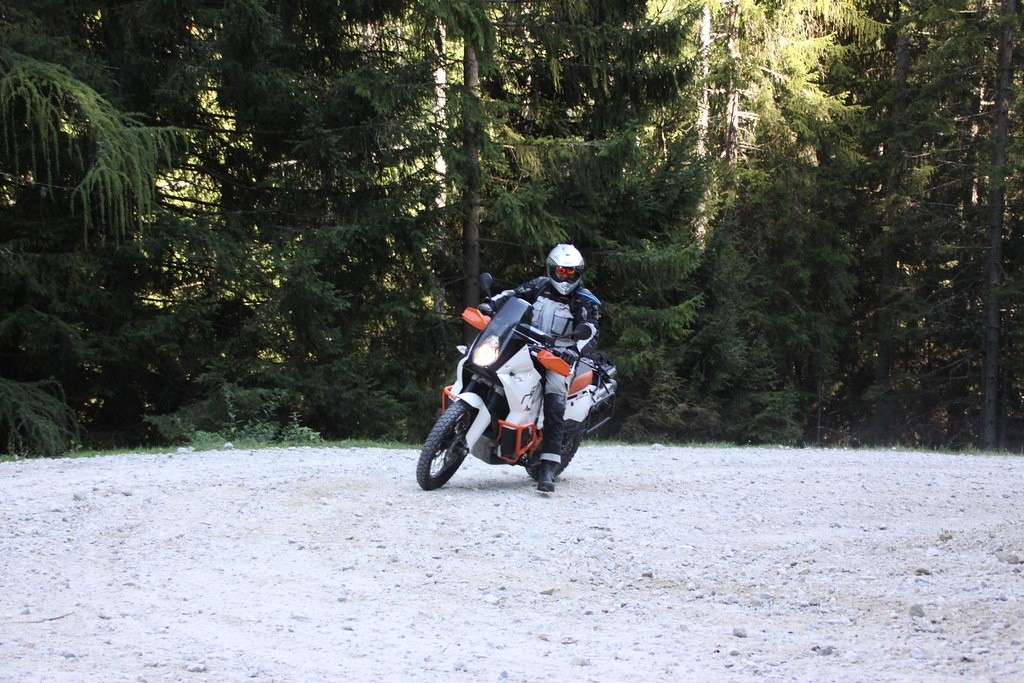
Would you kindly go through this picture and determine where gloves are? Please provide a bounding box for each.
[479,303,493,316]
[560,348,579,366]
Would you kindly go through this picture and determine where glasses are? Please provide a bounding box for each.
[556,266,575,275]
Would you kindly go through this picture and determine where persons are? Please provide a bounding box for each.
[480,244,600,493]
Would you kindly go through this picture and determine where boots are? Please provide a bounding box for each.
[536,453,561,492]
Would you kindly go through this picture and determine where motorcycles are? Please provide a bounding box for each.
[418,270,619,491]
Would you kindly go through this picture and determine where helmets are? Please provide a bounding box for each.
[545,244,584,295]
